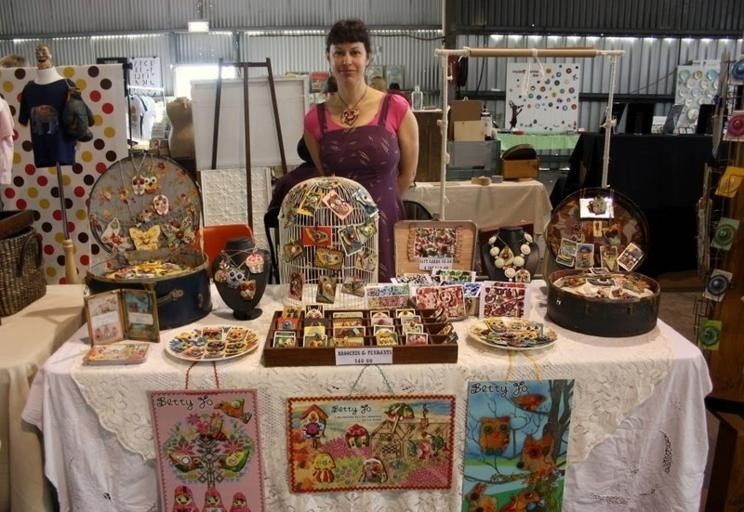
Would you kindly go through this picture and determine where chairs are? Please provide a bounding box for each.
[203,220,253,276]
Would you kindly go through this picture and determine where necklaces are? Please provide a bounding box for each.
[571,187,587,239]
[118,158,153,224]
[152,194,169,216]
[337,85,367,126]
[144,152,158,194]
[129,152,145,196]
[488,232,532,282]
[214,244,265,300]
[497,236,523,267]
[602,185,621,247]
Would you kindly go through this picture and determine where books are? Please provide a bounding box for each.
[81,343,151,364]
[82,288,160,345]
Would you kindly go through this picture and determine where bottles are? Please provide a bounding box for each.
[481,106,492,141]
[411,85,423,111]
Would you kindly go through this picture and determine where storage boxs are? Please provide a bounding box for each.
[542,188,662,341]
[392,219,483,319]
[445,97,539,180]
[81,150,214,330]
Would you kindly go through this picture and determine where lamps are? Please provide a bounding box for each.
[183,3,212,36]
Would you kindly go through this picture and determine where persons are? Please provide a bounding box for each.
[368,76,388,93]
[323,76,338,100]
[265,134,321,228]
[16,46,95,169]
[303,18,419,282]
[385,82,412,110]
[166,97,194,159]
[0,53,30,70]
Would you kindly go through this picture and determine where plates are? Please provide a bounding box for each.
[469,316,557,351]
[165,324,259,362]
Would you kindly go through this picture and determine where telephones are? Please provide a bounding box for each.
[458,58,467,87]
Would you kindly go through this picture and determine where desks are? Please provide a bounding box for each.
[410,177,555,255]
[18,280,718,509]
[495,130,582,171]
[0,279,91,511]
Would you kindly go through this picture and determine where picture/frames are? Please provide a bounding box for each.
[83,288,126,348]
[120,287,163,344]
[190,74,312,173]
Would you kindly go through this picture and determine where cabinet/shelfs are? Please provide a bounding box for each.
[406,107,447,182]
[575,130,718,277]
